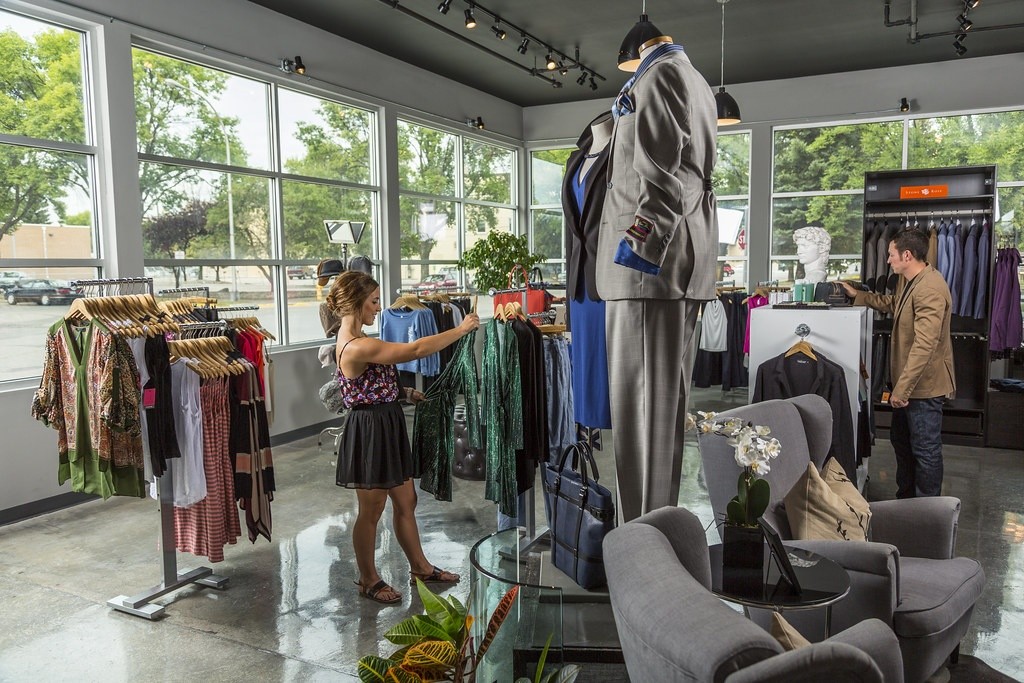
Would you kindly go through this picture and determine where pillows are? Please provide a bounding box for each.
[785,458,871,542]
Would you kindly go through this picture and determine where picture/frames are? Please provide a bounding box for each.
[765,524,804,590]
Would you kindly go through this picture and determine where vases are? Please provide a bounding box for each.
[718,520,764,563]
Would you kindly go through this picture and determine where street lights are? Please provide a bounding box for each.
[165,77,241,302]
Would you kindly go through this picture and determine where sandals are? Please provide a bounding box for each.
[358,579,402,603]
[410,566,460,585]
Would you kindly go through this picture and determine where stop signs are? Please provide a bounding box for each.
[738,229,747,250]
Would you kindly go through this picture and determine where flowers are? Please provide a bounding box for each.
[681,409,780,527]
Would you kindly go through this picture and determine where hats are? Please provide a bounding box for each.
[317,260,345,285]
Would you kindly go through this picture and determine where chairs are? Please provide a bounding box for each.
[604,393,985,683]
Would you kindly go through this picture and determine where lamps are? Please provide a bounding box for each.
[714,0,741,126]
[469,117,486,130]
[283,55,307,74]
[899,96,908,112]
[953,0,979,56]
[439,0,599,91]
[618,0,663,72]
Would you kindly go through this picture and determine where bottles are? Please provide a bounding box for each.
[804,279,814,303]
[793,279,803,302]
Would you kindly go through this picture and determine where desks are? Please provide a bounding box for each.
[708,543,852,641]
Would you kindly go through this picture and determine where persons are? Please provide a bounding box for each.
[592,34,719,523]
[324,271,478,604]
[791,226,831,285]
[561,109,613,430]
[835,231,957,499]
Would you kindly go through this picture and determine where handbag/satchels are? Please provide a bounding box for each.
[494,265,545,326]
[527,267,554,313]
[544,440,615,589]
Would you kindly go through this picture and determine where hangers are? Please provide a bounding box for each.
[999,232,1021,259]
[383,289,545,338]
[52,279,276,377]
[707,283,790,305]
[785,335,818,361]
[865,208,995,237]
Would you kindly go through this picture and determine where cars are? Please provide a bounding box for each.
[4,279,86,307]
[287,265,315,278]
[437,267,471,284]
[410,274,459,300]
[0,269,26,293]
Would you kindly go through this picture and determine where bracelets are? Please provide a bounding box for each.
[405,387,414,403]
